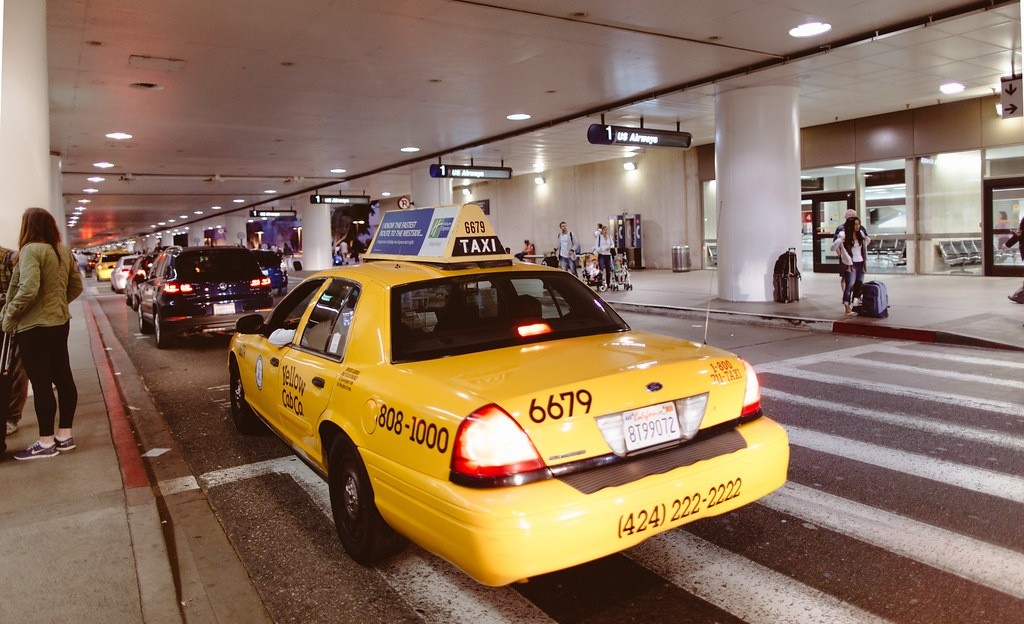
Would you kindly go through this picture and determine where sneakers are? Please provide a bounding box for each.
[54,436,77,451]
[14,440,60,461]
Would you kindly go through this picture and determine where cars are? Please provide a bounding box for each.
[249,249,288,295]
[125,253,160,311]
[95,251,129,281]
[109,255,141,293]
[227,204,790,587]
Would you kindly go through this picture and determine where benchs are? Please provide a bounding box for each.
[937,239,1014,266]
[868,239,906,260]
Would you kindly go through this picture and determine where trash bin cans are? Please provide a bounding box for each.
[672,245,691,273]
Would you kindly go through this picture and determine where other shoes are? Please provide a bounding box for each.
[6,421,19,435]
[0,443,7,454]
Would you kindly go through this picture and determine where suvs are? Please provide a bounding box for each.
[135,244,274,347]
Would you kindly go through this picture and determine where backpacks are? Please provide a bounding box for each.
[774,252,801,279]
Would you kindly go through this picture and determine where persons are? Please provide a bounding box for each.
[0,207,86,461]
[995,211,1017,264]
[260,243,295,271]
[332,238,372,265]
[514,222,626,285]
[830,209,871,316]
[1003,216,1024,304]
[131,242,163,256]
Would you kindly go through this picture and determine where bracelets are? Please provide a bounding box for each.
[554,248,557,251]
[570,249,575,252]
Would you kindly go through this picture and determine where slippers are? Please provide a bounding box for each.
[845,311,858,316]
[1008,293,1024,303]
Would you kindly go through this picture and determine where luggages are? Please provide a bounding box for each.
[861,283,887,313]
[541,252,559,290]
[773,248,800,304]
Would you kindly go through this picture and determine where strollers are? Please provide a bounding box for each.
[579,252,607,292]
[610,248,632,291]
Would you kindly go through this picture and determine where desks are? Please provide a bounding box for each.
[522,254,581,265]
